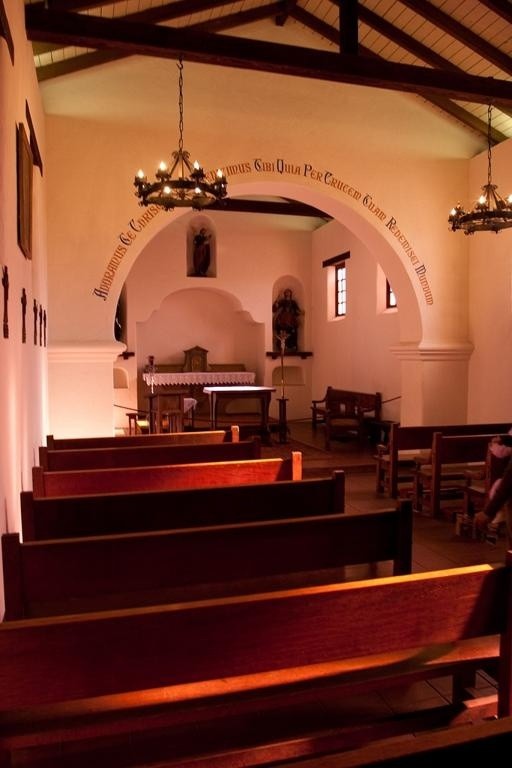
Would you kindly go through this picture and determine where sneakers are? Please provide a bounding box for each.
[488,521,506,529]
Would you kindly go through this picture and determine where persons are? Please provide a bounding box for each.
[271,288,302,352]
[187,227,211,277]
[114,296,124,342]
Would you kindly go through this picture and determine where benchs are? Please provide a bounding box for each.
[370,423,512,546]
[32,426,301,500]
[1,469,412,621]
[1,548,511,768]
[309,386,385,452]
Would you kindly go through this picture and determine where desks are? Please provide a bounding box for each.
[143,370,277,434]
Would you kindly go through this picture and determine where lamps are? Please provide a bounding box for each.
[447,93,512,235]
[131,59,227,210]
